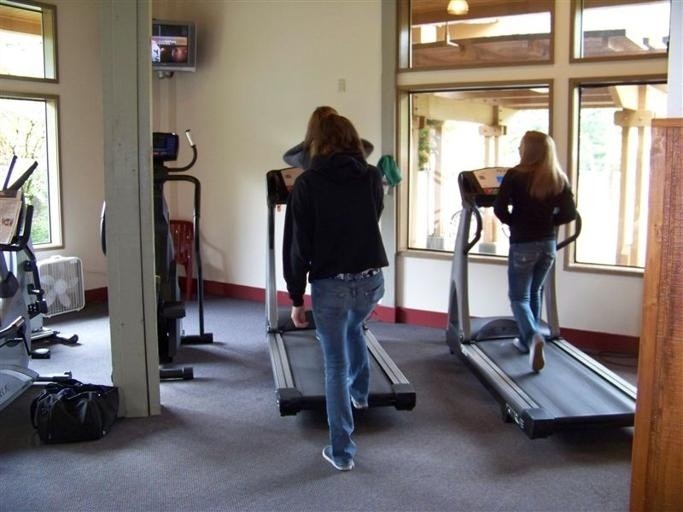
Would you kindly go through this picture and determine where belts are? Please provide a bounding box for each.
[328,267,383,282]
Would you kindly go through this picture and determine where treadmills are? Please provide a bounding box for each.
[265,167,416,416]
[447,167,638,438]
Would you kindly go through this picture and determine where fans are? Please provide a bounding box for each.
[35,256,86,319]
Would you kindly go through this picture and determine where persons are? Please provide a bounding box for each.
[494,131,576,373]
[282,106,374,342]
[280,113,388,470]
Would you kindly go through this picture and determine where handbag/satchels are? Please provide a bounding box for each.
[33,376,118,445]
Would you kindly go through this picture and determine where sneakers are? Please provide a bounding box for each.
[349,394,368,410]
[322,445,353,471]
[529,334,545,372]
[512,338,529,354]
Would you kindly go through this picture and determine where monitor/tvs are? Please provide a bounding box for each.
[152,20,197,73]
[153,132,179,161]
